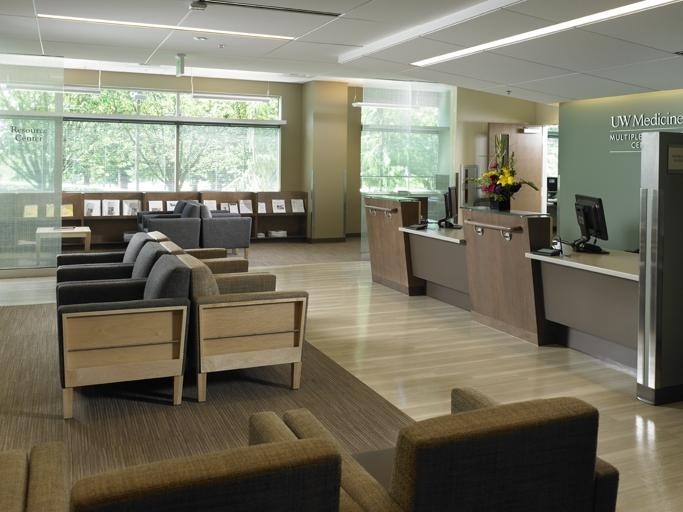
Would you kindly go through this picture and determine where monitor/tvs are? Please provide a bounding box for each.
[437,186,462,229]
[547,176,558,199]
[571,194,609,254]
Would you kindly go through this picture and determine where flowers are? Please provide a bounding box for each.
[462,140,540,201]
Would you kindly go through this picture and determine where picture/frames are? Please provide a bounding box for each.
[489,194,511,214]
[494,134,509,169]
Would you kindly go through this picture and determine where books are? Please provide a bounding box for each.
[21,197,306,218]
[268,230,287,238]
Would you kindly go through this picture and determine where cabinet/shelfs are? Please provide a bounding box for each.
[143,194,200,211]
[0,194,15,250]
[365,195,428,296]
[82,195,143,248]
[256,193,309,240]
[14,194,83,251]
[200,193,255,241]
[460,205,553,346]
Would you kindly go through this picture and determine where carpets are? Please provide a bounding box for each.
[0,303,416,481]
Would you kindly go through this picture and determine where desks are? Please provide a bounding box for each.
[34,225,91,265]
[524,242,639,352]
[397,223,470,311]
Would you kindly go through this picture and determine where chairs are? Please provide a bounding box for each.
[137,200,188,231]
[143,202,200,249]
[0,450,29,512]
[284,386,619,511]
[159,241,273,275]
[56,232,155,265]
[176,253,307,402]
[188,199,240,215]
[57,253,190,419]
[195,202,252,259]
[26,409,366,511]
[57,241,168,285]
[147,230,244,258]
